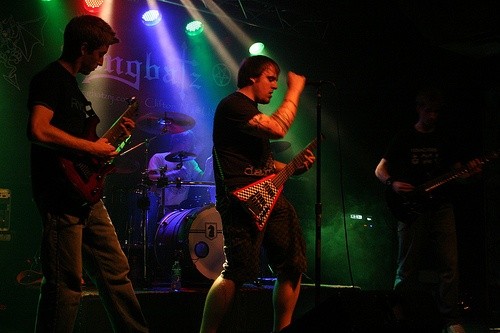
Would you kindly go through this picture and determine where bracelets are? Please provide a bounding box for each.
[386,178,395,185]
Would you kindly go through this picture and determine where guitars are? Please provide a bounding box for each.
[53,95,140,205]
[230,131,327,234]
[382,149,500,226]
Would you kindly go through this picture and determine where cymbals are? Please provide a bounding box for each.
[269,139,292,154]
[165,151,198,162]
[136,111,194,135]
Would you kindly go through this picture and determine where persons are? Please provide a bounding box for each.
[375,96,481,333]
[147,129,213,285]
[200,56,316,333]
[27,14,148,333]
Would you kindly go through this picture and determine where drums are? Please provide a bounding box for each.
[154,203,226,284]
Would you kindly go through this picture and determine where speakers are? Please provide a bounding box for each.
[279,286,443,333]
